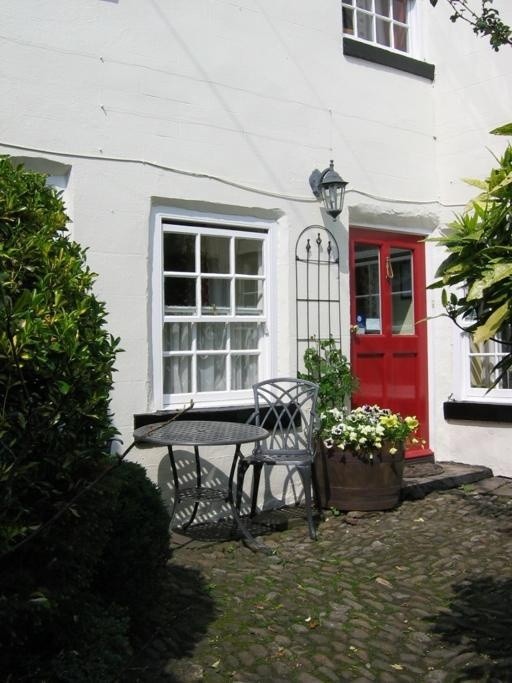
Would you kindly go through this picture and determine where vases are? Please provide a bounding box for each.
[314,443,405,512]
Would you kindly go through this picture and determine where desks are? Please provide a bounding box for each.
[135,421,270,555]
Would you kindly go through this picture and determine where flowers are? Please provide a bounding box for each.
[294,334,427,452]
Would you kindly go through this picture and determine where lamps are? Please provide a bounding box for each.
[309,159,348,222]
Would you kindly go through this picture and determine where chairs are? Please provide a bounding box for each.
[227,377,320,540]
